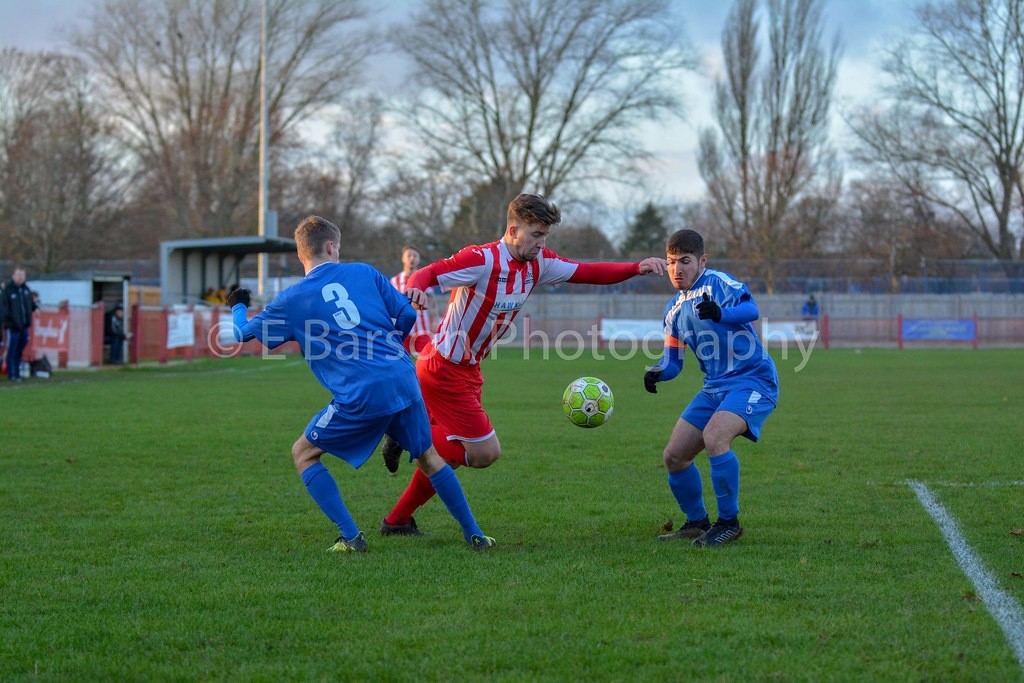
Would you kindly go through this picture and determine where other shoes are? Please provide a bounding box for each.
[9,376,23,383]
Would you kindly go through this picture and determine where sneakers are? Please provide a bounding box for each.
[324,531,368,553]
[472,534,497,551]
[657,514,711,543]
[381,435,404,473]
[691,516,743,547]
[380,516,431,535]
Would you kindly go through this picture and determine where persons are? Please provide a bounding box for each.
[101,300,134,364]
[802,294,821,318]
[643,229,779,549]
[2,268,36,382]
[205,286,240,307]
[379,195,668,534]
[388,245,434,355]
[226,214,495,551]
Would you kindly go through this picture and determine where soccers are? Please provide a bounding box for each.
[562,376,615,429]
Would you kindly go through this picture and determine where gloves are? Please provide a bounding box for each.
[694,292,721,324]
[643,366,662,395]
[227,288,252,308]
